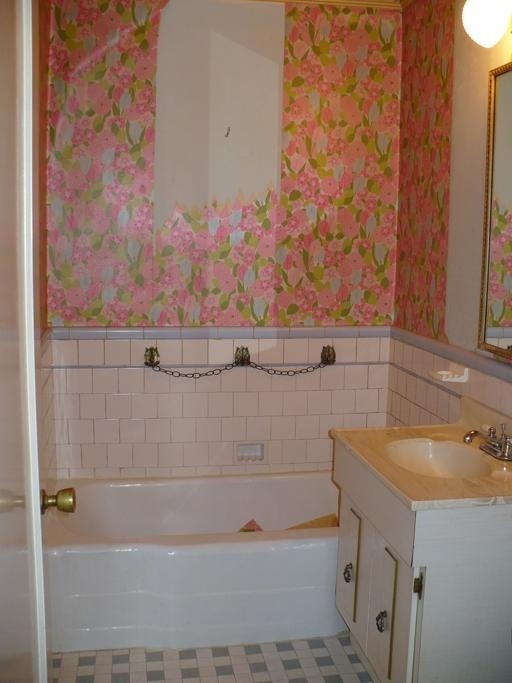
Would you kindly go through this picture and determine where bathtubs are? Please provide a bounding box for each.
[42,471,345,651]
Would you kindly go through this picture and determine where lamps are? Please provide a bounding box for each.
[462,0,512,50]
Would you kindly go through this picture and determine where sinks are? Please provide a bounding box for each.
[386,438,493,479]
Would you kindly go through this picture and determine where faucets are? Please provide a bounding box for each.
[463,428,499,452]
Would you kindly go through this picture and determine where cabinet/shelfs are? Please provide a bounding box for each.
[335,489,512,683]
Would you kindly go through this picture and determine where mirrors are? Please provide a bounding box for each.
[477,62,512,361]
